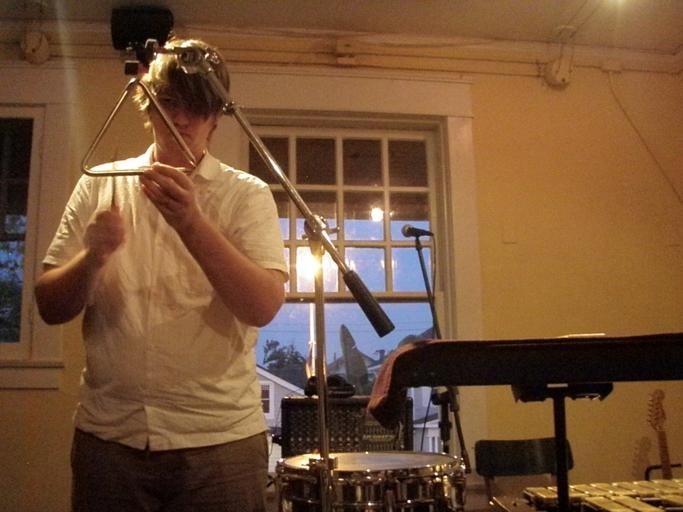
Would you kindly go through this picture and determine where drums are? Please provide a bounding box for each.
[276,449,465,510]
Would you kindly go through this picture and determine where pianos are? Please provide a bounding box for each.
[395,330,682,389]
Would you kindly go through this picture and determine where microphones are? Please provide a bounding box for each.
[401,224,434,237]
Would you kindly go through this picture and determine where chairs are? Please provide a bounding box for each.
[475,437,572,503]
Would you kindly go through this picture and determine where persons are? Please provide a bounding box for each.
[32,35,290,512]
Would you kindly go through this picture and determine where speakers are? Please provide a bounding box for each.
[110,6,174,49]
